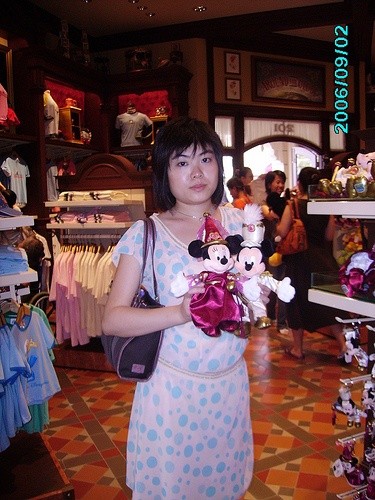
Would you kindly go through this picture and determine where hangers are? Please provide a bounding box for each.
[9,145,18,156]
[1,290,30,328]
[59,234,118,253]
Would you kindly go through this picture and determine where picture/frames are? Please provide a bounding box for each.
[225,51,241,75]
[250,55,326,108]
[225,77,242,101]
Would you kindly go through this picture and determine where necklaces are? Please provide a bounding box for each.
[172,206,215,222]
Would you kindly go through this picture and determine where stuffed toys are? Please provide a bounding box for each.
[320,154,375,500]
[171,203,294,338]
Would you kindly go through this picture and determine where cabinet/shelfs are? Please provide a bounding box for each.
[58,106,83,144]
[307,183,375,319]
[43,199,142,229]
[0,216,39,286]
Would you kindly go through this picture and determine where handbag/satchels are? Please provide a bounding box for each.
[275,198,307,255]
[102,218,165,381]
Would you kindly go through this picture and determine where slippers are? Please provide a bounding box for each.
[335,339,352,359]
[284,346,304,362]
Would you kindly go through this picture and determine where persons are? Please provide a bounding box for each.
[16,226,47,309]
[225,167,347,358]
[43,90,59,136]
[101,116,270,500]
[115,107,152,148]
